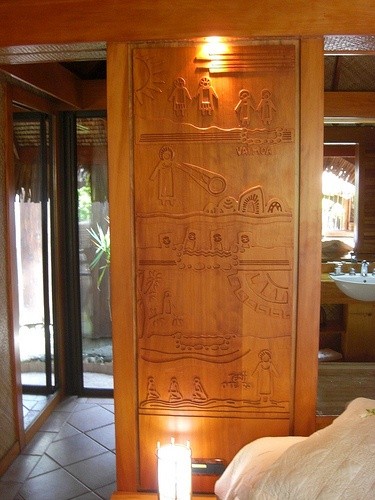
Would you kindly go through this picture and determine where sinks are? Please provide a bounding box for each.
[329,273,375,302]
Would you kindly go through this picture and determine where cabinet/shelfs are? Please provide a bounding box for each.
[320,270,375,360]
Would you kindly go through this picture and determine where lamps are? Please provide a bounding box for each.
[157,439,194,500]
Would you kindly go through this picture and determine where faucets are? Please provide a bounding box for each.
[360,261,370,277]
